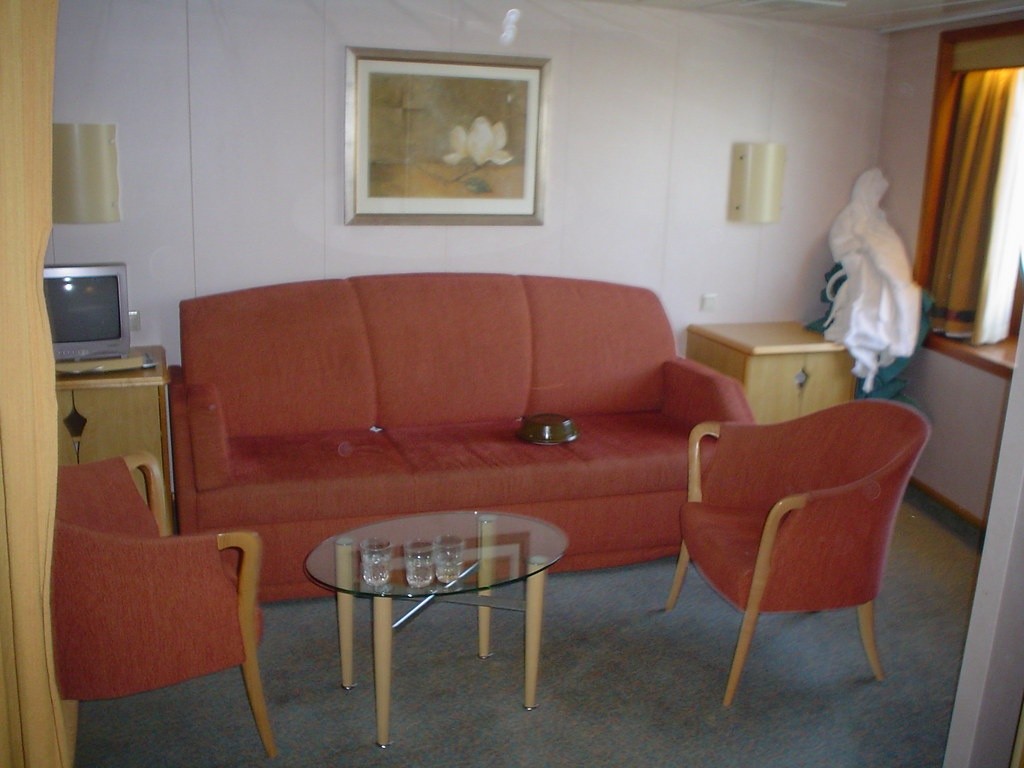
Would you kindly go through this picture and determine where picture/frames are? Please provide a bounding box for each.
[344,44,551,226]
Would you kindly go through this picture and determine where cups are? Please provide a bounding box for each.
[434,534,464,583]
[403,536,435,589]
[360,536,392,587]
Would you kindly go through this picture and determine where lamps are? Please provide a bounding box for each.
[50,123,123,222]
[728,142,787,222]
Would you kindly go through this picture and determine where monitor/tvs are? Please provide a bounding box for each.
[43,262,131,359]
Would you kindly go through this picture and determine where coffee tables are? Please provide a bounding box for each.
[303,509,568,750]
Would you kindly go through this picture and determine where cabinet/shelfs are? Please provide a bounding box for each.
[684,323,857,426]
[54,342,173,510]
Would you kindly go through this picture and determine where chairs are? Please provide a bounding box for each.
[661,400,931,711]
[52,456,277,758]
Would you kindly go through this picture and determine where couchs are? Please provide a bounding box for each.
[164,273,758,605]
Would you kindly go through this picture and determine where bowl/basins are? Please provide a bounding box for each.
[518,413,580,442]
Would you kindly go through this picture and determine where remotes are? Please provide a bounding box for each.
[142,352,156,369]
